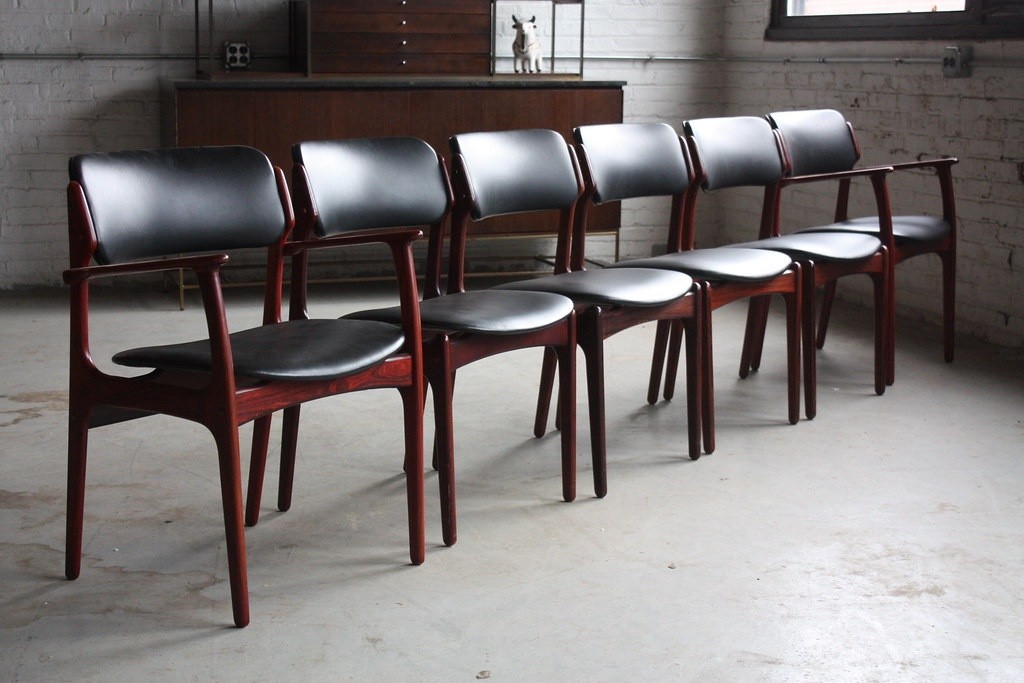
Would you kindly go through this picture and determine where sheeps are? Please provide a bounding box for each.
[511,14,542,73]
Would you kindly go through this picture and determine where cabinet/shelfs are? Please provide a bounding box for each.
[290,0,497,78]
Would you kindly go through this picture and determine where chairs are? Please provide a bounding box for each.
[531,121,803,455]
[278,135,576,546]
[662,115,890,419]
[66,145,429,628]
[433,126,701,504]
[751,107,962,386]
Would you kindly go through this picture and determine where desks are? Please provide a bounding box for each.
[155,72,627,290]
